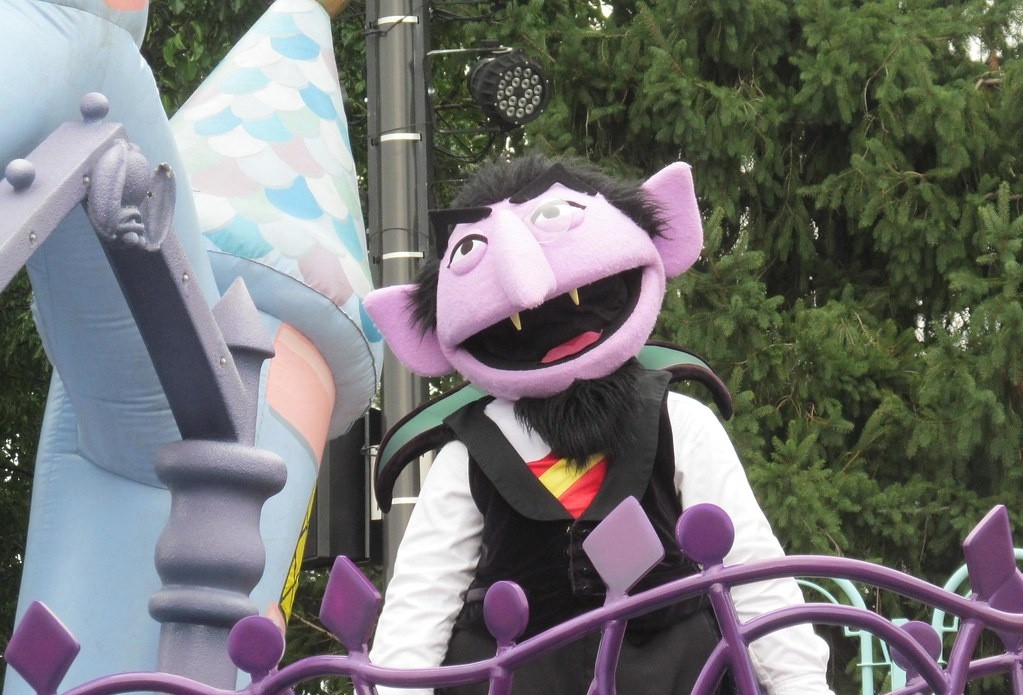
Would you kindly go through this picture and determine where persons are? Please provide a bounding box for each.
[363,155,835,695]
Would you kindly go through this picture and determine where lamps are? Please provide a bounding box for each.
[470,47,552,126]
